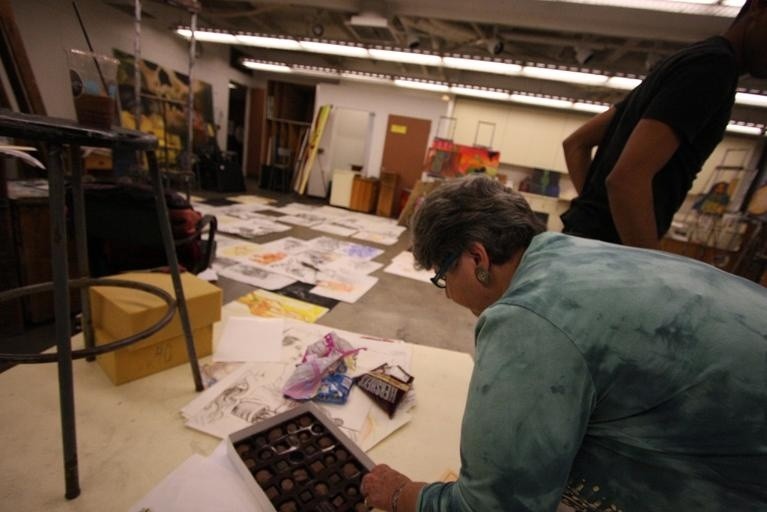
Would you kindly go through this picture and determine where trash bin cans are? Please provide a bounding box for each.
[349,175,379,213]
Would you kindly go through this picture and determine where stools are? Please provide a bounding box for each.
[1,99,210,510]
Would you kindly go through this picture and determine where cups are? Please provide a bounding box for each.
[66,47,120,132]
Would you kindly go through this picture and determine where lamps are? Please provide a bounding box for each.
[724,85,765,138]
[176,23,644,92]
[450,83,614,113]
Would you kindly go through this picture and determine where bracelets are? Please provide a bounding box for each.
[392,475,411,511]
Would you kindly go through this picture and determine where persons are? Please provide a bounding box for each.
[360,173,765,512]
[689,179,732,220]
[560,0,767,250]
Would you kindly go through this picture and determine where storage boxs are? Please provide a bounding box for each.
[83,265,227,351]
[85,321,219,391]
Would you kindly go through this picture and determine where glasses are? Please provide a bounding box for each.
[430,242,475,289]
